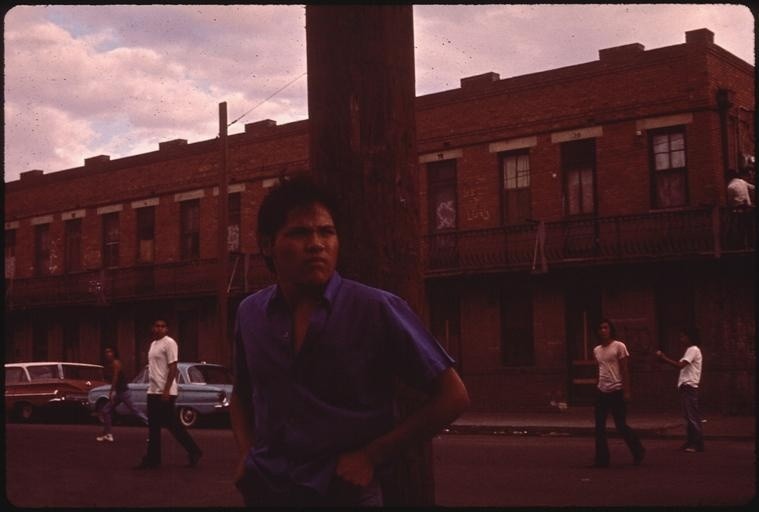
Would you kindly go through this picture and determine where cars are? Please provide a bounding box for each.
[87,360,233,426]
[4,362,105,419]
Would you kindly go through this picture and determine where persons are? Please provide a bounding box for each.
[725,170,756,212]
[592,318,647,468]
[656,326,703,453]
[131,316,202,470]
[96,344,150,442]
[229,179,470,507]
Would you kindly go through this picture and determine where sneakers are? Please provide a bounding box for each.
[96,434,114,442]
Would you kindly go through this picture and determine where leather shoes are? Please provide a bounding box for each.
[131,456,161,469]
[190,451,203,465]
